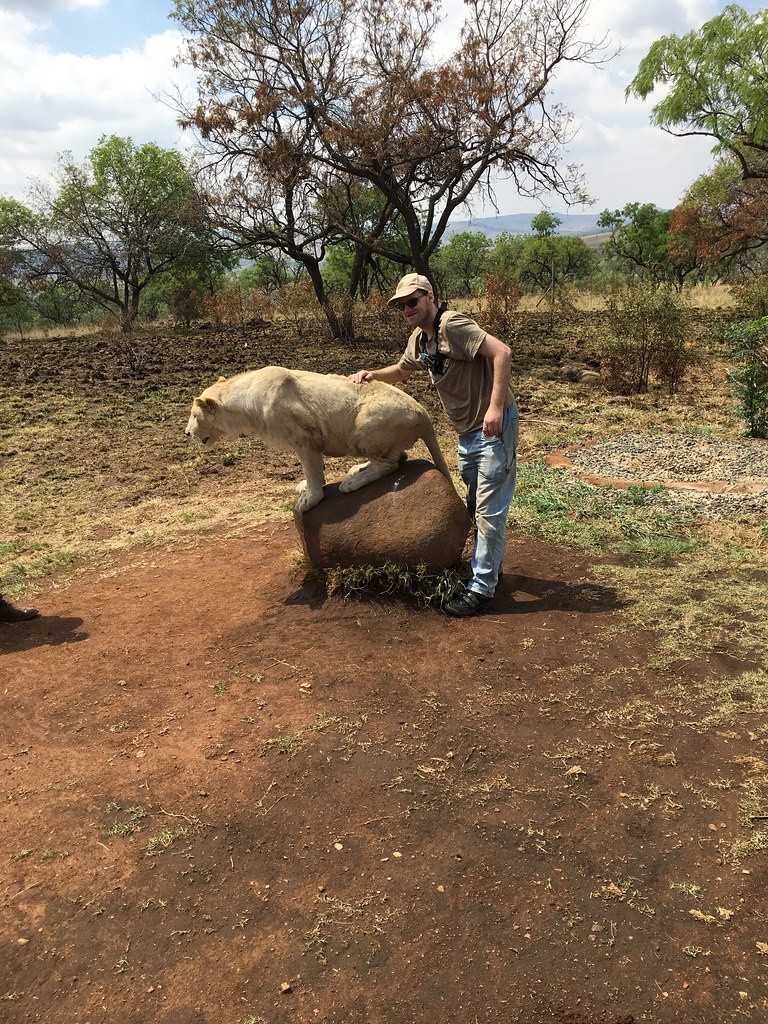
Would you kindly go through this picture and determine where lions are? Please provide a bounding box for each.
[184,366,456,514]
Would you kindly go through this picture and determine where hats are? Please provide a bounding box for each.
[387,273,433,308]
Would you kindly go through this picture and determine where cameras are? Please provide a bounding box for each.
[420,353,435,366]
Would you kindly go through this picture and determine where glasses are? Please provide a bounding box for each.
[394,294,427,312]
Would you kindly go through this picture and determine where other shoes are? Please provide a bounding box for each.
[444,589,494,616]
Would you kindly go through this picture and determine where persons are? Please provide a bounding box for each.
[0,594,39,621]
[347,274,519,617]
